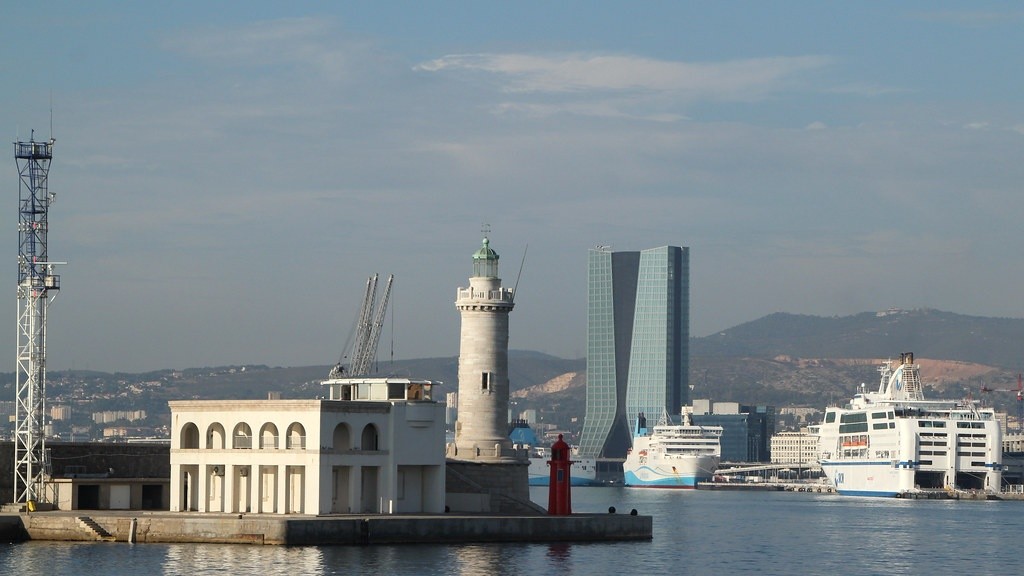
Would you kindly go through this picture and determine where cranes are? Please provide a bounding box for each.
[348,271,394,376]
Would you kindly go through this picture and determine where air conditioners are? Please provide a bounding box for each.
[213,466,224,475]
[240,469,247,476]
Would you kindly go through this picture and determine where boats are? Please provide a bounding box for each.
[622,406,723,489]
[816,351,1003,499]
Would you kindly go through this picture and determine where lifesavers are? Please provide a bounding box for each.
[807,488,812,492]
[817,489,821,492]
[799,487,805,492]
[895,492,901,499]
[827,489,832,493]
[791,487,794,491]
